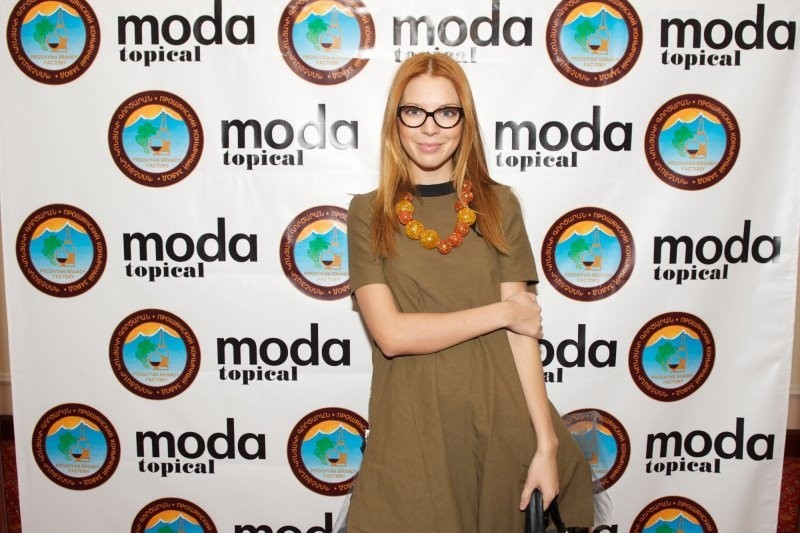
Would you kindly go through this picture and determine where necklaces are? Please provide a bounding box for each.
[395,175,477,255]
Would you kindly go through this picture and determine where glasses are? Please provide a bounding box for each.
[397,105,464,129]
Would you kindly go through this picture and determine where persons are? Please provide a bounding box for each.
[329,52,612,533]
